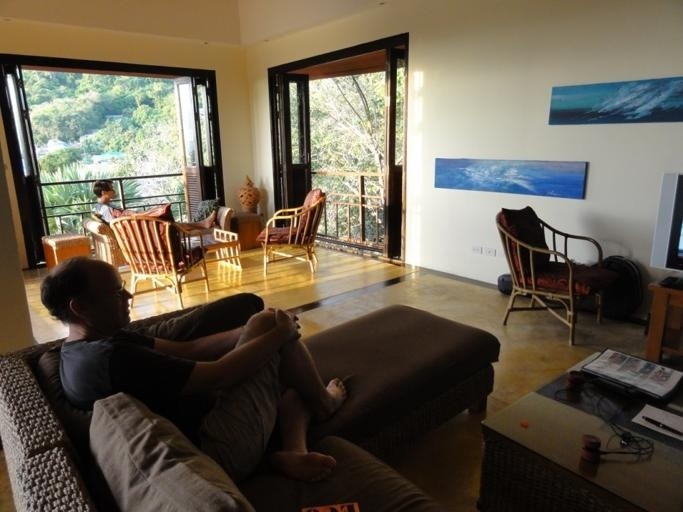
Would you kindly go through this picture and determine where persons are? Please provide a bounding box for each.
[41,258,346,482]
[91,179,216,236]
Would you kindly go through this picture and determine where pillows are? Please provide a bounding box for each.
[500,206,552,272]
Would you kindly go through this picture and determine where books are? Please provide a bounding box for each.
[582,348,681,399]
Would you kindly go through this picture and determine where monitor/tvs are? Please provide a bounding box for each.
[650,173,683,291]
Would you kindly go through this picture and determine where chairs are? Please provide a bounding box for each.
[78,185,328,313]
[495,205,617,348]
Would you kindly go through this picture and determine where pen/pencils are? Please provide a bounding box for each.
[641,415,683,436]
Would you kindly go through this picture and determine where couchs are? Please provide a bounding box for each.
[0,294,443,511]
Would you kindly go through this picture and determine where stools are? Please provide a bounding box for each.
[41,233,91,268]
[298,302,500,450]
